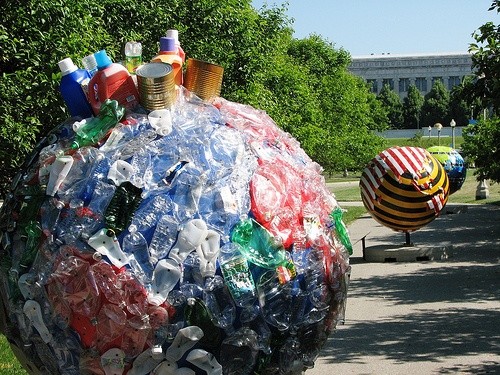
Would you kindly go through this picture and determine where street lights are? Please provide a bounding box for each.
[436,123,442,146]
[449,119,456,149]
[475,73,490,199]
[428,126,431,138]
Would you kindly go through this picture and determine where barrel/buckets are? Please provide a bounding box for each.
[135,62,175,112]
[184,56,223,102]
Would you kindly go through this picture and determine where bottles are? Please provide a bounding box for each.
[82,54,99,78]
[151,28,185,88]
[1,88,355,375]
[56,57,95,118]
[88,48,141,116]
[124,42,142,87]
